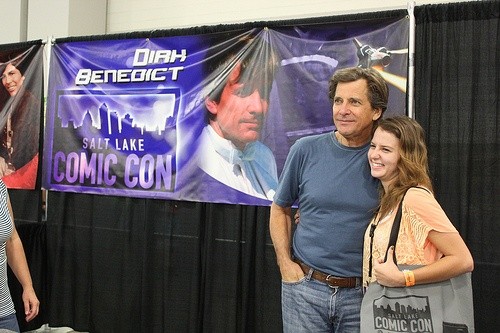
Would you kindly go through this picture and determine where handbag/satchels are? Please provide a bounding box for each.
[359,185,475,333]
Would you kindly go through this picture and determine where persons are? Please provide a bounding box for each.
[194,32,282,201]
[269,65,389,333]
[362,115,474,296]
[0,179,40,333]
[0,52,41,179]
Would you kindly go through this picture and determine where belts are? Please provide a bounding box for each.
[291,257,363,287]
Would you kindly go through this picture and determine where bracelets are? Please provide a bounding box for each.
[402,269,415,287]
